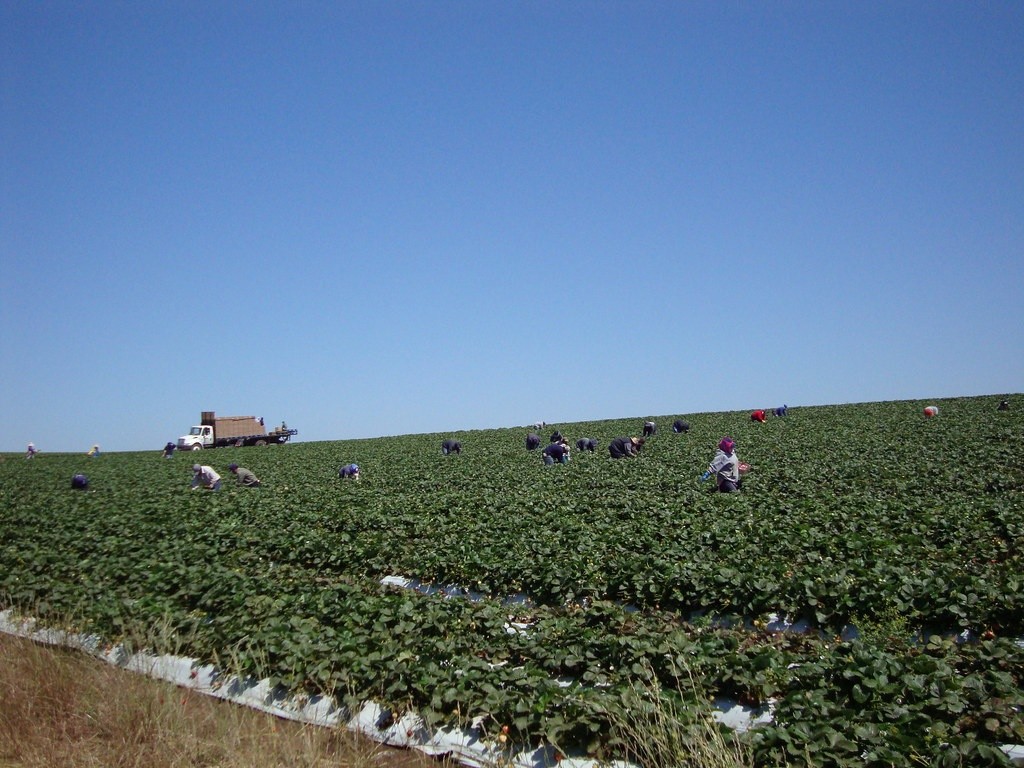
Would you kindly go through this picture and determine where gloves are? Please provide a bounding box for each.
[703,472,709,479]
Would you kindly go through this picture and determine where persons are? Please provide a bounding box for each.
[28,444,36,459]
[924,404,939,416]
[282,420,287,430]
[339,464,359,479]
[576,438,598,453]
[256,416,264,425]
[192,464,221,490]
[610,437,645,458]
[643,422,656,437]
[525,421,571,466]
[776,404,787,416]
[751,409,766,422]
[72,474,89,492]
[164,442,177,459]
[230,464,259,487]
[442,440,461,455]
[673,420,689,433]
[92,445,100,457]
[699,438,745,494]
[998,401,1008,410]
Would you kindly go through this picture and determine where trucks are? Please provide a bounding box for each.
[176,411,297,451]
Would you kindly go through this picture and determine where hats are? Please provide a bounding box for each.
[193,464,200,476]
[352,463,359,472]
[565,445,570,454]
[229,464,238,472]
[562,437,569,442]
[630,437,638,444]
[591,439,597,446]
